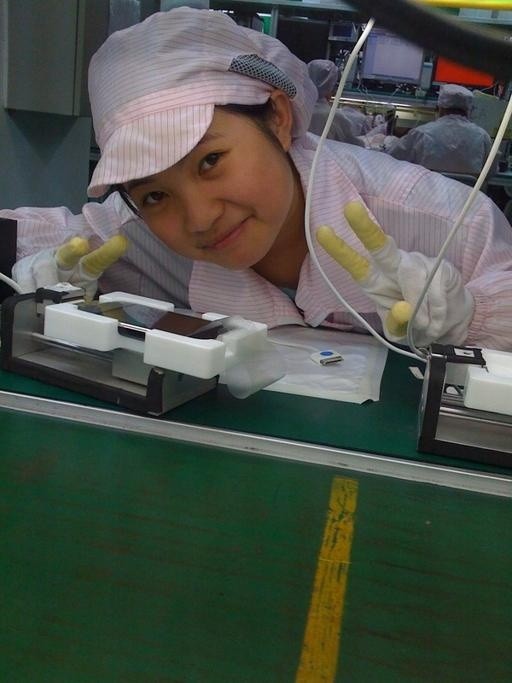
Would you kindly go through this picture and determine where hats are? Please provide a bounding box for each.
[306,59,339,96]
[81,5,319,205]
[436,84,477,114]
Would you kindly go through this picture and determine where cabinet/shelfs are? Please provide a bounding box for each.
[4,0,109,117]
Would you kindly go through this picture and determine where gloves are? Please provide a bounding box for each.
[10,234,128,308]
[315,199,475,356]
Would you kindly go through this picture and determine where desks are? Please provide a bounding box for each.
[0,326,512,683]
[487,170,512,216]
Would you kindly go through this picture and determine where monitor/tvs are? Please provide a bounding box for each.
[357,24,426,96]
[427,47,497,101]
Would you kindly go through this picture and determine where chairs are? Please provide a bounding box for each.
[430,168,487,193]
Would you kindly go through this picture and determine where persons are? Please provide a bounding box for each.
[0,6,512,352]
[383,84,498,180]
[307,59,353,141]
[339,81,372,136]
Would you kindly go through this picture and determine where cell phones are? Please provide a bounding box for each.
[78,299,222,339]
